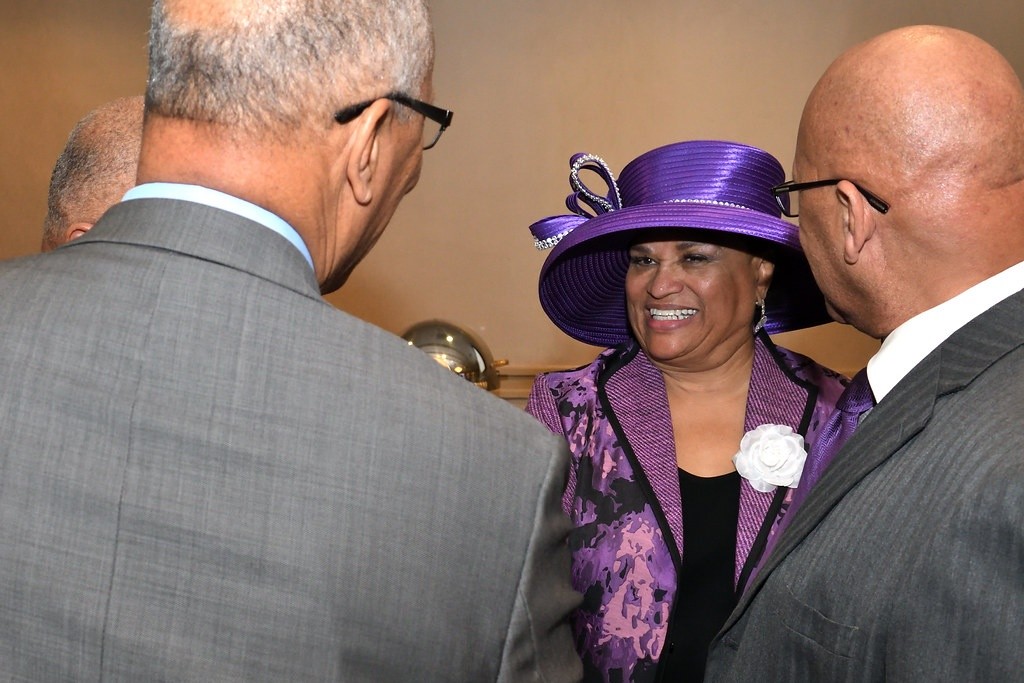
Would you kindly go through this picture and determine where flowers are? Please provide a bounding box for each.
[732,423,808,493]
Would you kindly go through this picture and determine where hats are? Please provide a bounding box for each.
[529,142,840,349]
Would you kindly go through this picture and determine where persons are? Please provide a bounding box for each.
[521,139,853,683]
[0,0,585,682]
[705,22,1023,682]
[40,93,146,253]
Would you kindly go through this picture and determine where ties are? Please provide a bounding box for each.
[787,365,875,530]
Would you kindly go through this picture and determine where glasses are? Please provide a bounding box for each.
[769,180,890,216]
[333,91,454,151]
